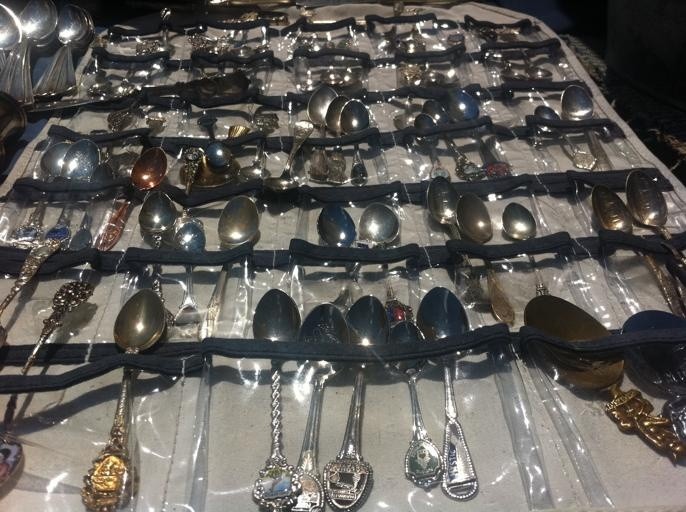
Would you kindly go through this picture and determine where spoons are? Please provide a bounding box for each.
[384,303,444,491]
[80,289,169,512]
[294,303,345,512]
[521,295,684,466]
[623,309,684,404]
[1,8,684,290]
[417,287,480,501]
[251,291,304,510]
[324,295,388,510]
[1,282,92,493]
